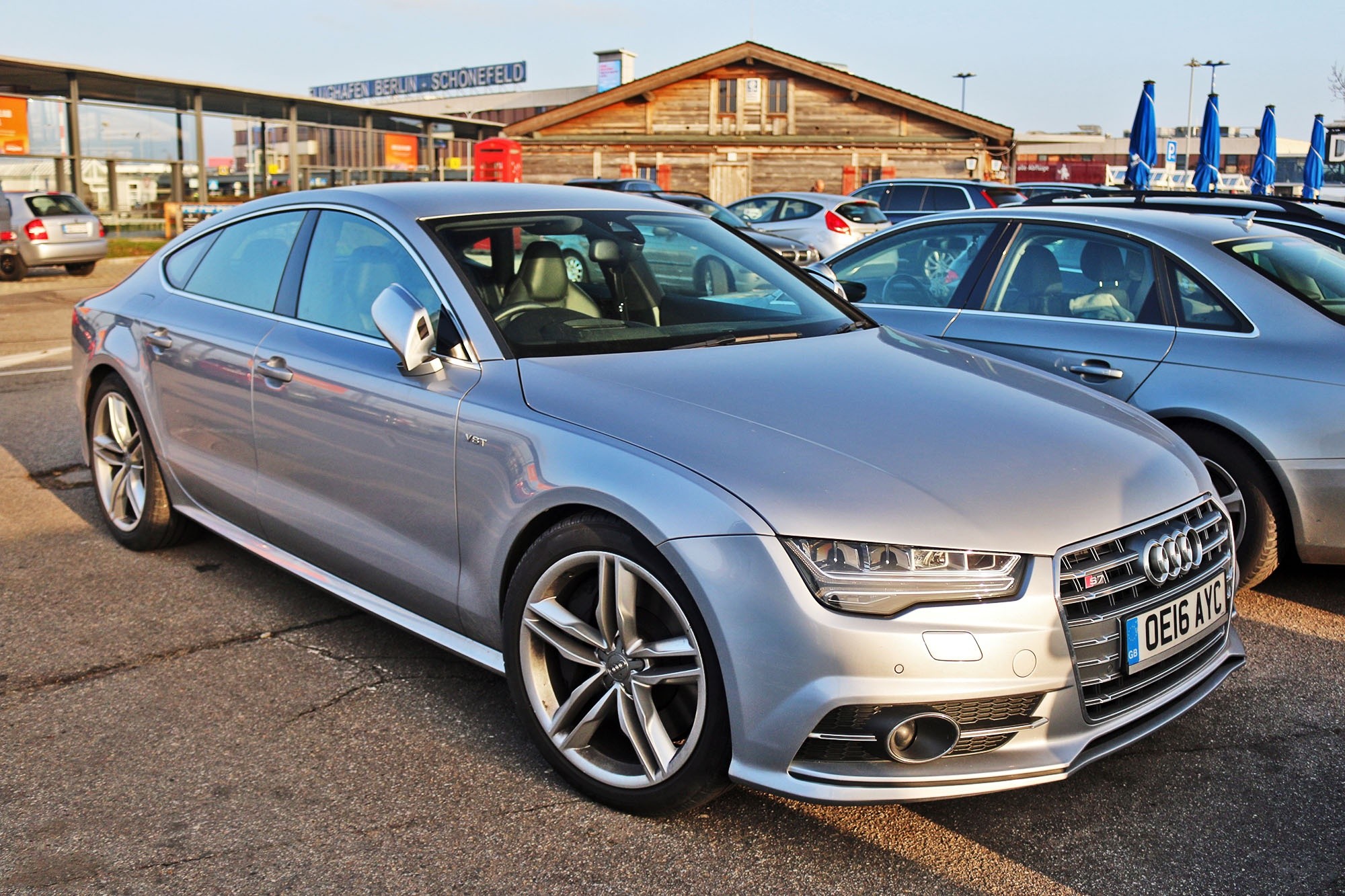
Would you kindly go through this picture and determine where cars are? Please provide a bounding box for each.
[67,177,1250,821]
[0,186,108,283]
[808,204,1345,591]
[560,174,1345,272]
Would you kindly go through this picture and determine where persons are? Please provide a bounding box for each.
[804,179,824,215]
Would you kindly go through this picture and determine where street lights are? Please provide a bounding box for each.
[953,71,977,112]
[1182,56,1201,190]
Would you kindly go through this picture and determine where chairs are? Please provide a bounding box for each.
[47,205,61,215]
[1077,243,1134,318]
[505,241,601,314]
[997,245,1061,313]
[338,243,467,336]
[784,207,795,217]
[241,238,290,298]
